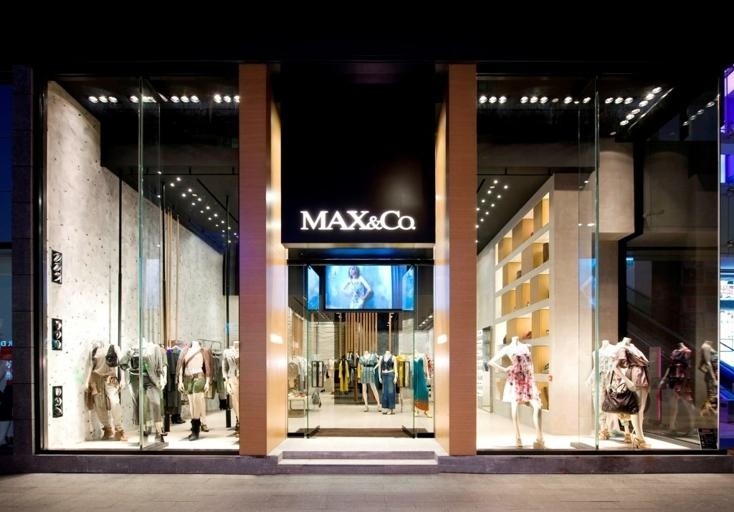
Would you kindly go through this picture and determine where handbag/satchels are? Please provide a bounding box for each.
[603,389,639,414]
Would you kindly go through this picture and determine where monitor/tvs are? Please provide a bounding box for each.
[322,264,409,312]
[401,265,417,312]
[305,264,320,311]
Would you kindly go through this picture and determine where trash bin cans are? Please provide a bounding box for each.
[699,426,717,449]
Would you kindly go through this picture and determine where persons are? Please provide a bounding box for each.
[488,336,544,447]
[586,337,718,449]
[84,340,240,441]
[410,352,432,418]
[343,266,372,308]
[359,351,381,411]
[378,351,398,414]
